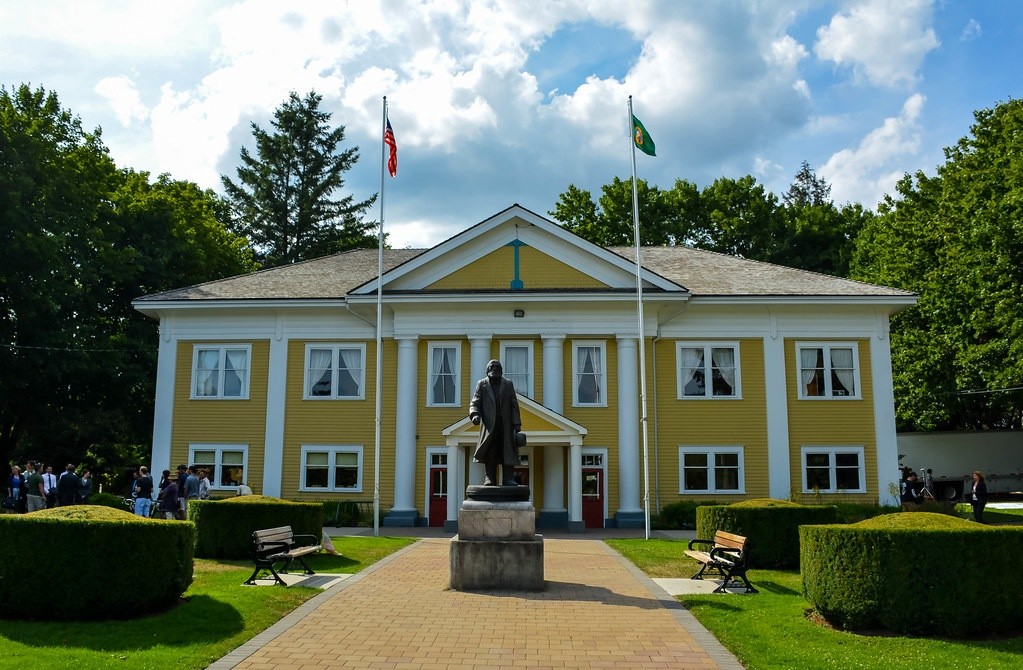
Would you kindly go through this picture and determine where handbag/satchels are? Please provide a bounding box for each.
[3,495,17,510]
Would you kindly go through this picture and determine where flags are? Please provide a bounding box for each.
[632,115,657,157]
[384,118,398,177]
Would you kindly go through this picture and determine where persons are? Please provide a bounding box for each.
[514,472,522,485]
[236,483,253,497]
[900,469,934,505]
[963,470,988,523]
[131,464,210,521]
[6,461,93,514]
[469,359,527,487]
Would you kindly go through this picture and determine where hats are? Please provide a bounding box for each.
[176,464,188,470]
[168,474,177,479]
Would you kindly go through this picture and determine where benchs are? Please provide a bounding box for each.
[683,529,761,594]
[241,526,323,586]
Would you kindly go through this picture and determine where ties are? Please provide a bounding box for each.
[48,475,51,489]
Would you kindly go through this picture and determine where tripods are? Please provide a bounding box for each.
[917,468,937,502]
